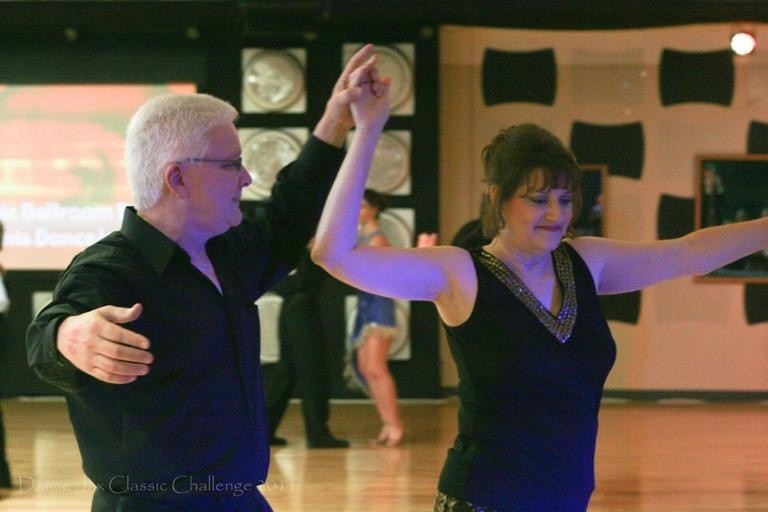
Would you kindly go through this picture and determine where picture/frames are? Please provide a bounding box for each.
[696,156,767,285]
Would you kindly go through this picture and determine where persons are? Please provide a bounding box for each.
[308,51,768,511]
[346,186,408,449]
[256,268,351,449]
[25,42,378,512]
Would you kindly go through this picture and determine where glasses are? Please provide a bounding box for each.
[178,157,241,170]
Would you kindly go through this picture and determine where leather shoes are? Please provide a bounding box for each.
[307,436,349,447]
[372,422,406,447]
[268,437,285,445]
